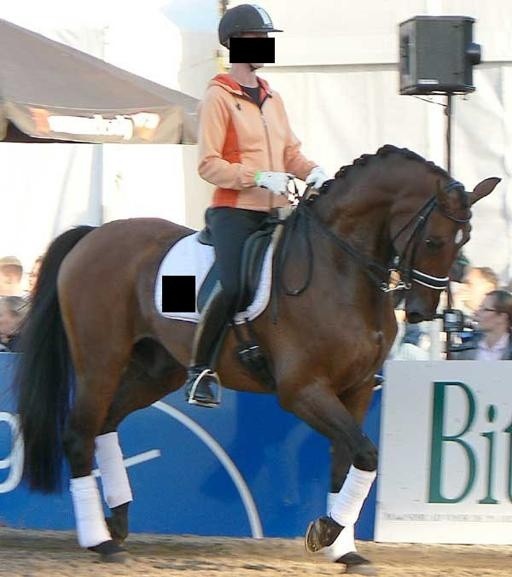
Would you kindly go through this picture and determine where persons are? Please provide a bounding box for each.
[186,3,327,402]
[386,265,511,361]
[0,253,44,353]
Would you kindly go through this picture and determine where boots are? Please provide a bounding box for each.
[184,280,241,402]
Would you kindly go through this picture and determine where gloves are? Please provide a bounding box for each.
[254,171,295,196]
[304,167,330,191]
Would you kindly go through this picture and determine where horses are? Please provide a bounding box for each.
[1,143,502,576]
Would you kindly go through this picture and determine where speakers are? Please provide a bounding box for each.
[399,16,479,89]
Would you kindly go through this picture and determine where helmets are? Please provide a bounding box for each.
[218,5,283,45]
